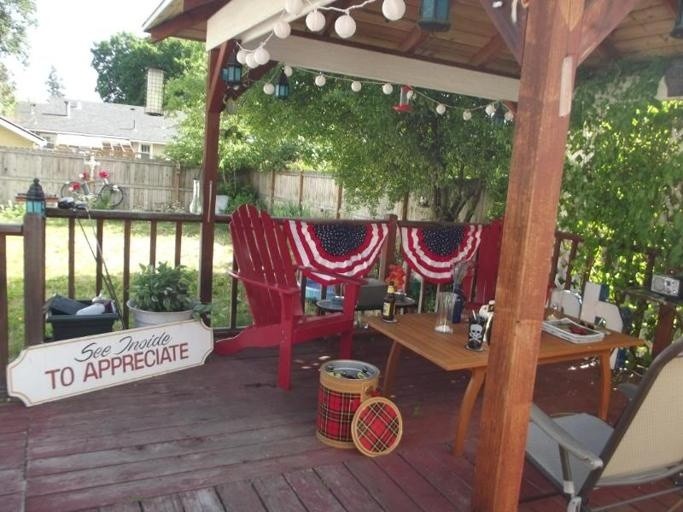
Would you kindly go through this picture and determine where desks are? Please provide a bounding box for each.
[362,307,646,458]
[315,295,417,316]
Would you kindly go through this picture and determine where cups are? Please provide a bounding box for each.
[469,315,489,351]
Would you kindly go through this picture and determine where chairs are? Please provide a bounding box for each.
[524,336,683,512]
[459,220,557,311]
[214,203,369,392]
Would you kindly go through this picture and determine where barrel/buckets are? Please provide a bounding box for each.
[315,359,403,459]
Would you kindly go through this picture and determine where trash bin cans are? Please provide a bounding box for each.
[315,359,382,454]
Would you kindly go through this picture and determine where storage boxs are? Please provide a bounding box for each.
[46,299,120,340]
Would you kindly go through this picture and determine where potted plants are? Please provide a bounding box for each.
[125,261,212,329]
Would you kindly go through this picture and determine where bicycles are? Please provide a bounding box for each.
[58,168,124,209]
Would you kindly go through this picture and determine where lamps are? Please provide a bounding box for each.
[418,0,451,33]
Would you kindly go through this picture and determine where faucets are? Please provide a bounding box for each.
[564,287,583,322]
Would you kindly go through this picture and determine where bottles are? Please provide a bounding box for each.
[328,364,369,379]
[382,280,395,323]
[76,303,106,316]
[433,281,456,335]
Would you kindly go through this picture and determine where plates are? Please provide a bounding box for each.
[543,317,606,344]
